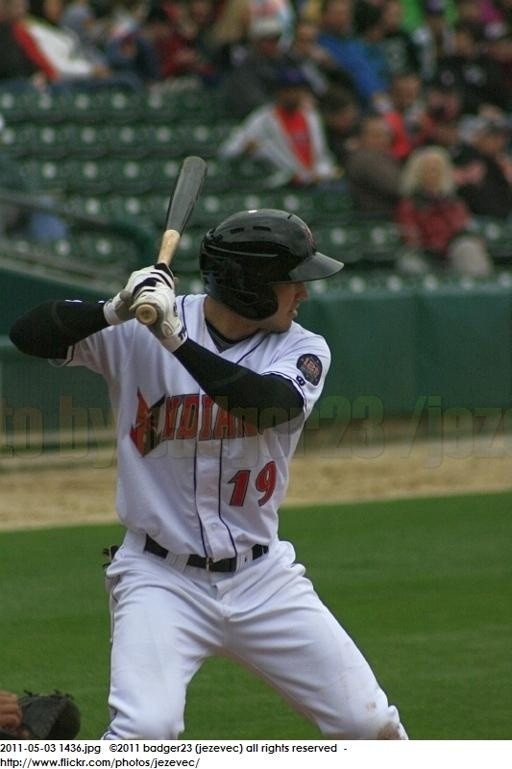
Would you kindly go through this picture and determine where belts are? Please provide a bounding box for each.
[143,533,268,572]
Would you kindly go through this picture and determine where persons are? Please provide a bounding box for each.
[7,207,416,741]
[0,682,82,740]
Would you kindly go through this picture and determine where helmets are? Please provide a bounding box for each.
[199,208,345,322]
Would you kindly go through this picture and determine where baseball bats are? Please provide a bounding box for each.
[130,156,206,325]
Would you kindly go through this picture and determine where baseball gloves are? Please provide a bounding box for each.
[1,689,80,740]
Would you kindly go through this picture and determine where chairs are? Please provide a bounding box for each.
[0,67,512,299]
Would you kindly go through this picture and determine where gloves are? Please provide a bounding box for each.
[103,262,187,352]
